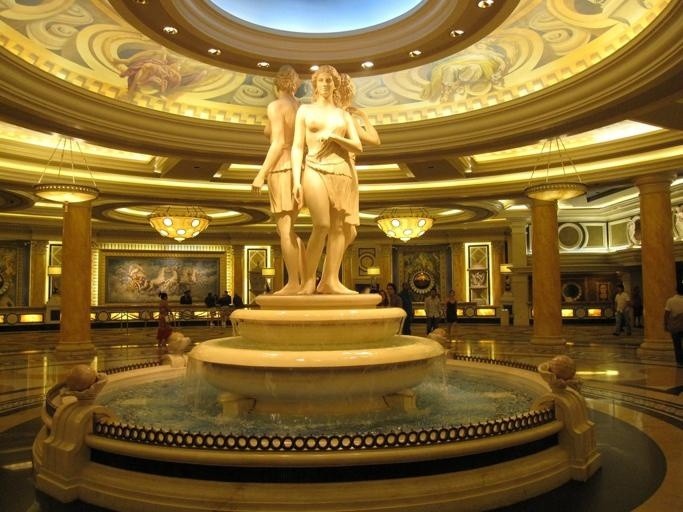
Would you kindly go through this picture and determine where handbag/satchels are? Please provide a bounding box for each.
[165,315,176,323]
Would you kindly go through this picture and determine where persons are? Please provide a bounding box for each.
[612,284,632,337]
[444,290,458,339]
[423,288,442,336]
[156,290,174,348]
[663,282,683,368]
[631,285,644,329]
[248,62,383,297]
[376,290,388,306]
[387,283,401,308]
[397,280,413,336]
[179,289,242,327]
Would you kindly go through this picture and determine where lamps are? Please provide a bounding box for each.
[146,198,214,244]
[376,204,437,244]
[525,134,590,202]
[31,131,99,207]
